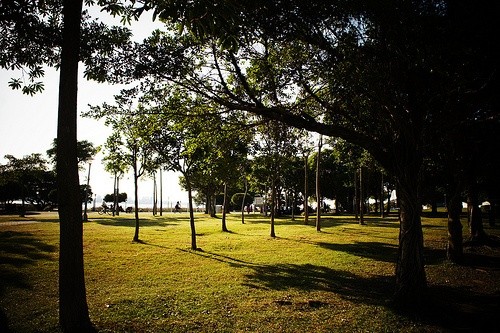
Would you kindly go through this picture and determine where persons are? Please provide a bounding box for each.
[175,200,181,211]
[246,202,250,214]
[102,202,109,214]
